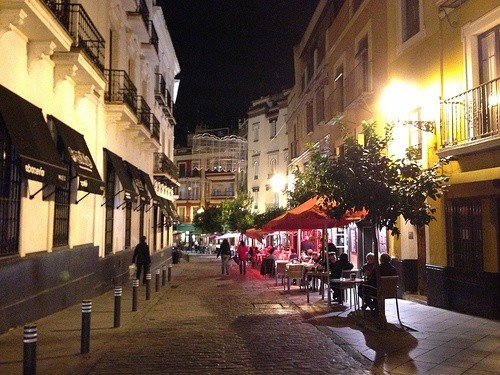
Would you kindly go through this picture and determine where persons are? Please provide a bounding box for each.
[358,252,397,311]
[194,239,199,254]
[132,236,151,286]
[216,239,231,276]
[236,241,248,275]
[248,243,354,303]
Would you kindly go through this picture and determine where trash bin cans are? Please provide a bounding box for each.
[172,247,180,264]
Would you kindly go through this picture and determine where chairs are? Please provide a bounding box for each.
[316,269,400,320]
[277,262,305,291]
[254,255,274,279]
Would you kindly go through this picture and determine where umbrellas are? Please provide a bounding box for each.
[245,193,370,240]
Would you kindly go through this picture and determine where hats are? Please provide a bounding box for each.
[328,251,337,254]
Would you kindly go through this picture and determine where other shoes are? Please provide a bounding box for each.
[244,272,247,275]
[143,283,146,286]
[240,271,242,274]
[222,272,224,274]
[365,302,376,309]
[227,273,229,275]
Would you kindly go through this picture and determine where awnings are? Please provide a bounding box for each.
[103,148,163,208]
[47,113,106,196]
[157,195,182,226]
[1,84,69,188]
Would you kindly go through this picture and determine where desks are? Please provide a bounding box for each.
[274,260,292,285]
[306,271,331,303]
[286,263,313,290]
[332,278,367,312]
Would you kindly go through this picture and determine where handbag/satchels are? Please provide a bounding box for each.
[225,250,230,254]
